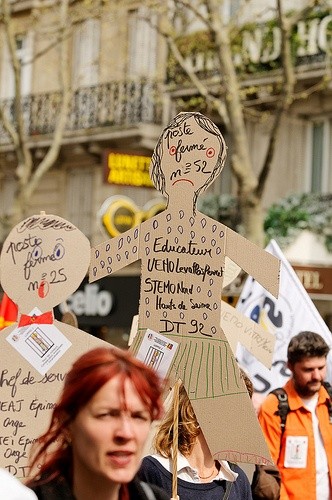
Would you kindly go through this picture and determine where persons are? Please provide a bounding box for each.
[22,344,180,500]
[256,331,332,500]
[134,364,256,500]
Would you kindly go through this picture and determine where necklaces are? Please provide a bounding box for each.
[198,463,217,479]
